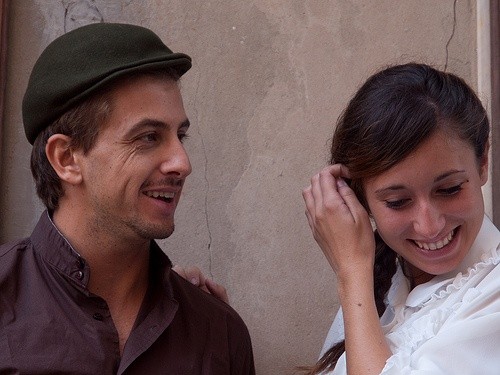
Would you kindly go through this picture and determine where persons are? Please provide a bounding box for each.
[171,61,500,375]
[0,23,256,375]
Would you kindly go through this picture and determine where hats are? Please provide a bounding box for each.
[22,23,192,145]
